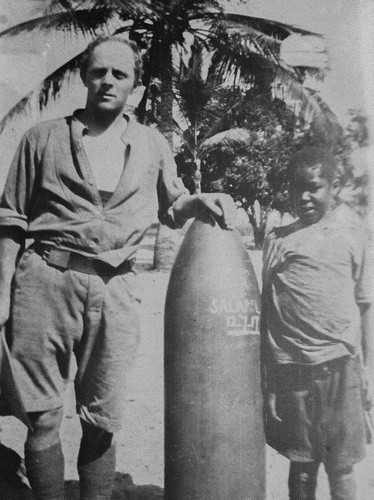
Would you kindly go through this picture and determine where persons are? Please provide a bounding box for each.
[255,144,374,500]
[0,35,242,499]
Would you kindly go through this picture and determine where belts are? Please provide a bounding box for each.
[34,242,133,277]
